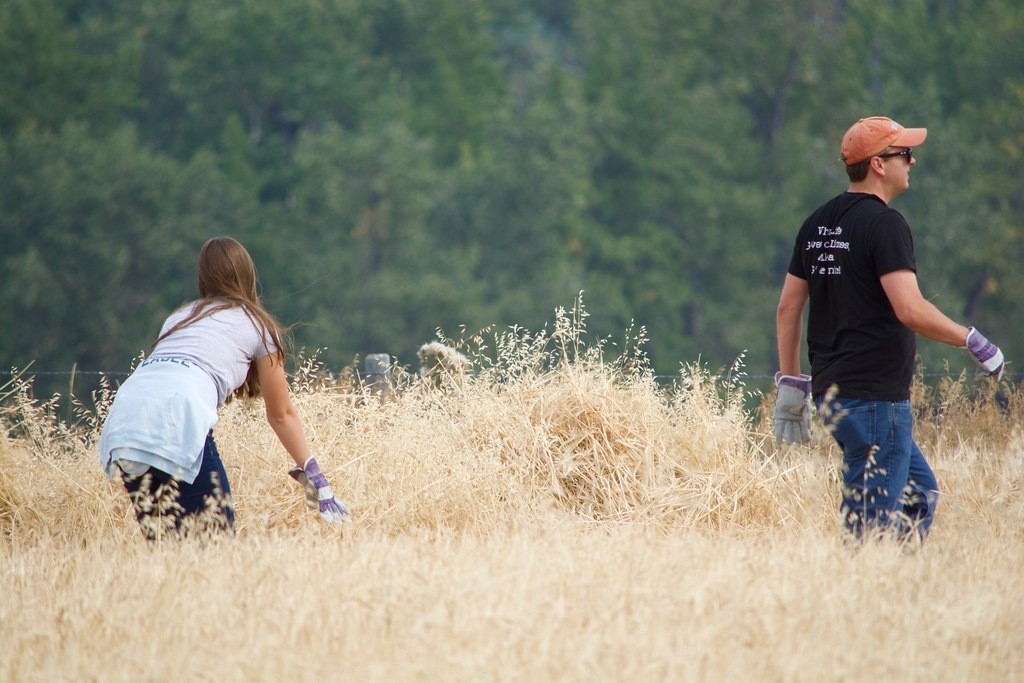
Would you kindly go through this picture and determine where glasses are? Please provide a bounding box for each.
[878,147,913,163]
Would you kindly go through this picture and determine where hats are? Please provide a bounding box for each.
[839,117,927,167]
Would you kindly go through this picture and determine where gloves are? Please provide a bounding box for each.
[287,455,352,523]
[774,371,812,447]
[953,326,1005,383]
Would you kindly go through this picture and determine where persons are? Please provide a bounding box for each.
[99,238,349,540]
[774,115,1005,546]
